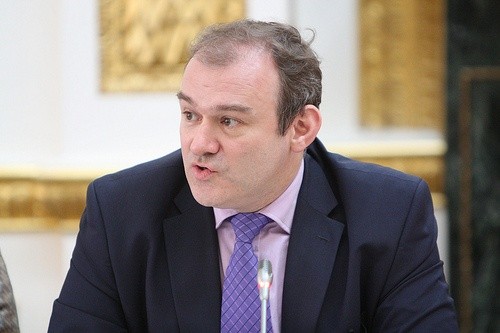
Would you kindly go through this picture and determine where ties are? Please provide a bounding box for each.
[221,213,275,333]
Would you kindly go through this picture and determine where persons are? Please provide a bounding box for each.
[46,19,460,333]
